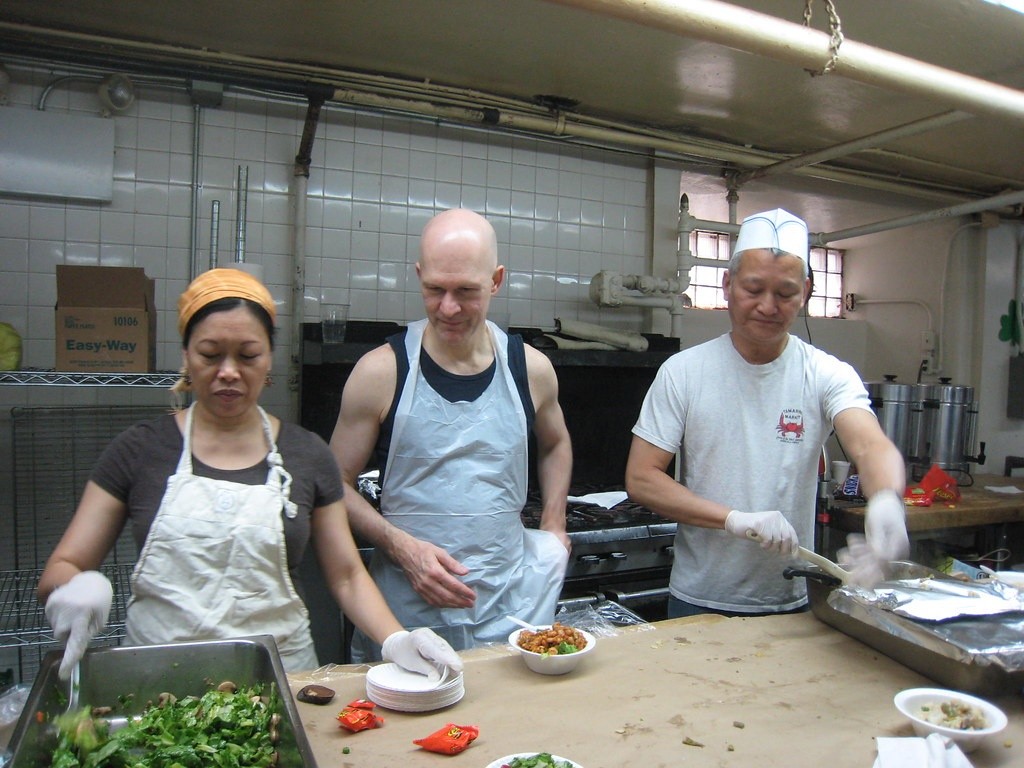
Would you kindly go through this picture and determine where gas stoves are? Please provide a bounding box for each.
[521,480,677,545]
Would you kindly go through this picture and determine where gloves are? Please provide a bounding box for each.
[865,489,910,563]
[725,510,799,559]
[45,570,113,680]
[381,626,463,682]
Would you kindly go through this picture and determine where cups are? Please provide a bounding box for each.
[320,302,351,343]
[832,461,850,484]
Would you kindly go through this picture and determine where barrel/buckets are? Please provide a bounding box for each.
[908,376,986,483]
[863,374,912,468]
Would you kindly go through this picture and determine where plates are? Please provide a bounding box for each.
[485,752,584,768]
[365,662,465,713]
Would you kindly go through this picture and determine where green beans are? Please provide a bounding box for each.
[518,622,586,654]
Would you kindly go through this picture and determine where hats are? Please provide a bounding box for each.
[734,208,809,266]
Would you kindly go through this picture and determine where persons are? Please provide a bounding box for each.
[625,207,911,619]
[36,267,465,685]
[328,207,573,665]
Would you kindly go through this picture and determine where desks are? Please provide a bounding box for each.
[822,474,1022,568]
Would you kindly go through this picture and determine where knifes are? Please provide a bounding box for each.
[782,566,901,589]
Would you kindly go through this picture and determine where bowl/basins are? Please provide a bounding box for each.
[0,634,316,768]
[893,688,1008,754]
[508,625,596,675]
[803,560,1024,698]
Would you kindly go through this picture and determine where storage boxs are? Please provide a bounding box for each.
[54,264,157,373]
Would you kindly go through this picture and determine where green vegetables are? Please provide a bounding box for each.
[49,681,278,768]
[506,752,574,768]
